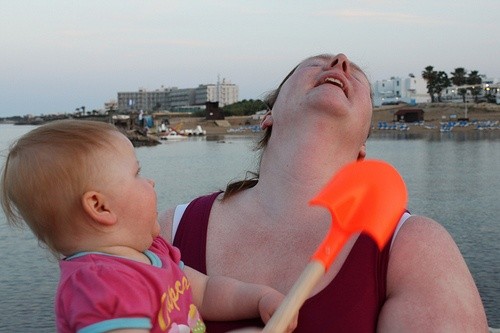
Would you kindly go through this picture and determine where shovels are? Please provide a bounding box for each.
[262,159,410,333]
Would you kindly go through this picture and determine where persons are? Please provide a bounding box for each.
[1,118,298,333]
[154,52,493,332]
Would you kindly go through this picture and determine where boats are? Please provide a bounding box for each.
[108,110,158,147]
